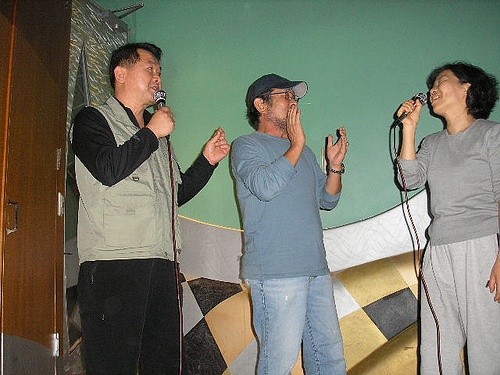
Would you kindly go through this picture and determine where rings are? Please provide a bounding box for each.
[346,142,349,146]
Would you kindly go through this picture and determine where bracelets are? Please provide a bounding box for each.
[326,163,345,173]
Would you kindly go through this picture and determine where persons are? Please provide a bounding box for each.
[397,62,500,375]
[231,73,349,375]
[69,42,230,375]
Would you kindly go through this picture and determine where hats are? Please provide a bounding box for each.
[245,73,308,106]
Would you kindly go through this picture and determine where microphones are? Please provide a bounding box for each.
[395,92,427,126]
[154,90,166,110]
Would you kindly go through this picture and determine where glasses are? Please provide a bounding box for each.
[261,91,299,102]
